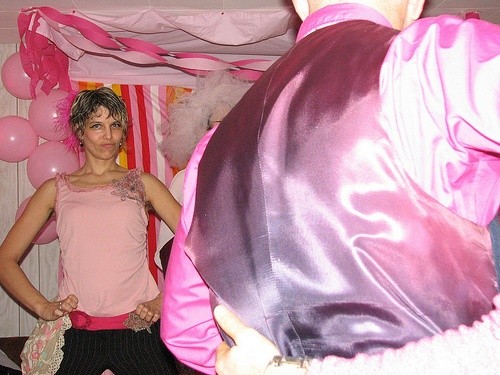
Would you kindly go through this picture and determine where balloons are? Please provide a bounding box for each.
[15,197,59,245]
[28,90,72,142]
[1,52,40,101]
[0,115,39,164]
[26,140,79,190]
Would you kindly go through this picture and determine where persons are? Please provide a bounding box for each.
[212,294,500,375]
[161,0,500,375]
[0,87,183,375]
[154,62,253,279]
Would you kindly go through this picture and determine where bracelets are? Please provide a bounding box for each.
[264,355,306,375]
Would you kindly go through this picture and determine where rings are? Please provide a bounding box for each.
[58,302,62,309]
